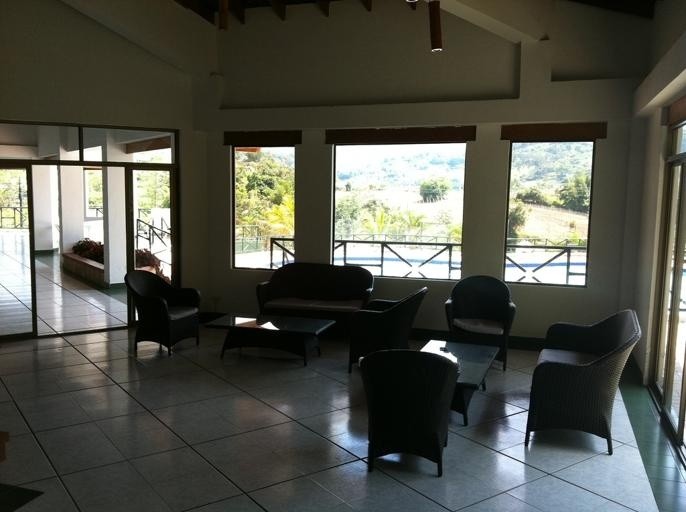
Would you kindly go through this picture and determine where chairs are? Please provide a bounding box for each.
[348,286,429,374]
[123,269,201,356]
[525,310,642,455]
[358,349,459,477]
[445,274,516,371]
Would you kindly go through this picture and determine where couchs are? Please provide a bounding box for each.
[256,263,373,337]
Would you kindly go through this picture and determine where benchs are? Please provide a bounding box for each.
[62,253,104,291]
